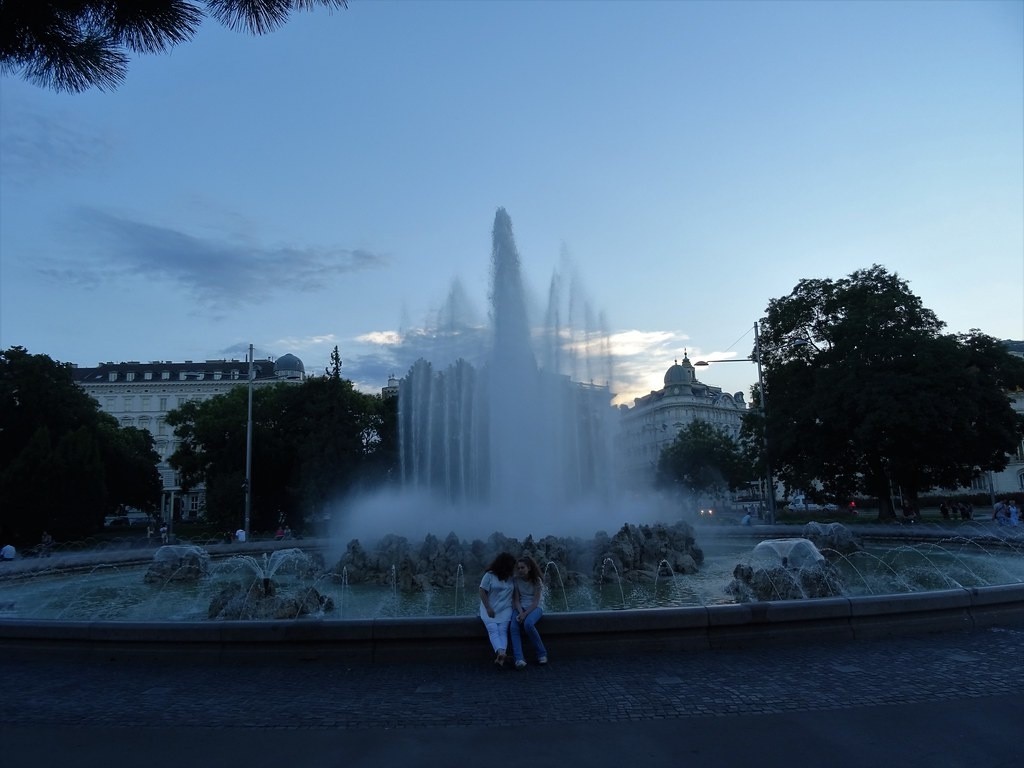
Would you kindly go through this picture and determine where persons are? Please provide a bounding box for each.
[40,530,52,558]
[273,524,292,540]
[0,541,17,562]
[223,526,246,544]
[741,502,758,526]
[901,499,918,524]
[847,494,857,513]
[510,555,548,669]
[478,553,517,668]
[992,497,1019,529]
[160,521,168,545]
[939,501,974,520]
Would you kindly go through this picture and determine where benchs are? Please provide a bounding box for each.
[192,540,217,545]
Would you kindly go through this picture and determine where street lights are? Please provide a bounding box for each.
[694,359,776,524]
[185,370,252,547]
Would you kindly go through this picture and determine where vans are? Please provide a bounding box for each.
[787,494,840,514]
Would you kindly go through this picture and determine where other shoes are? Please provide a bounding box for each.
[538,656,547,664]
[515,659,527,667]
[494,651,507,666]
